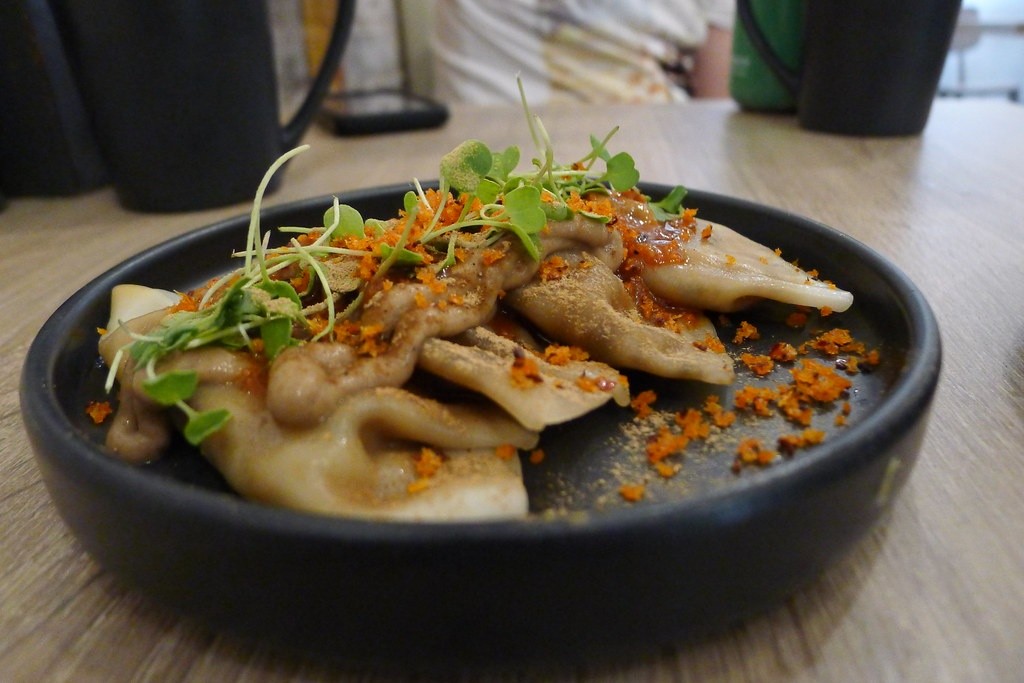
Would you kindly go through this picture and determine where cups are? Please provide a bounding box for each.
[0,0,449,215]
[728,0,963,136]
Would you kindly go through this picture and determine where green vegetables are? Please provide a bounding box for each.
[104,79,687,445]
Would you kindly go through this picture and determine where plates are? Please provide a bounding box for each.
[18,173,941,659]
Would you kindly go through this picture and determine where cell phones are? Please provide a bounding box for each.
[317,88,449,137]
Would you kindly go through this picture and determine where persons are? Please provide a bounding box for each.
[414,0,738,114]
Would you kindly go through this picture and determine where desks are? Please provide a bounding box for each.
[0,102,1024,683]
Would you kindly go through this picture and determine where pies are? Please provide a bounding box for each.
[102,217,853,520]
